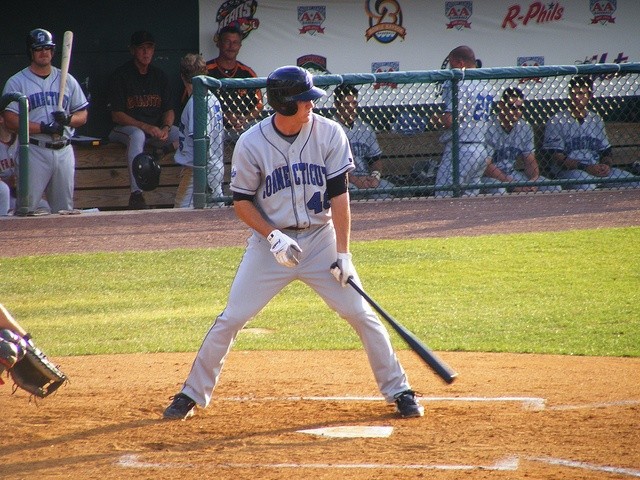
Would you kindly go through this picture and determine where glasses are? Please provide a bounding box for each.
[33,46,52,51]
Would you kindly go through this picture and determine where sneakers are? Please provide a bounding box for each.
[164,393,196,421]
[393,389,423,417]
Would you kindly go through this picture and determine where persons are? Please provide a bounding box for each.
[173,53,225,208]
[163,65,424,420]
[480,86,562,193]
[434,45,493,196]
[330,85,396,198]
[542,76,640,188]
[101,29,180,210]
[2,28,89,214]
[206,26,263,153]
[0,304,35,376]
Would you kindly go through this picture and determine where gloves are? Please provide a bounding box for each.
[40,120,65,136]
[333,252,354,288]
[266,230,302,268]
[8,333,71,408]
[51,111,72,125]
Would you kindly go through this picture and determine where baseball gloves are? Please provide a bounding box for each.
[0,328,27,375]
[9,338,67,399]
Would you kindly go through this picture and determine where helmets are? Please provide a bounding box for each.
[132,153,161,191]
[29,28,56,58]
[267,66,327,116]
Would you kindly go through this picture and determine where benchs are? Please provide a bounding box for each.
[73,122,640,211]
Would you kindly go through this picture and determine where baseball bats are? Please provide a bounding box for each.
[330,261,459,384]
[53,30,74,141]
